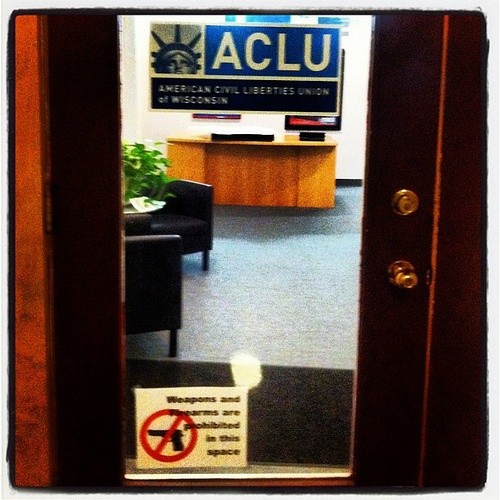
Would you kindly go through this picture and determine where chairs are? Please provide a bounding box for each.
[123,235,183,358]
[139,178,214,271]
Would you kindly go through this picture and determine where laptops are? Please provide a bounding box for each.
[286,114,341,130]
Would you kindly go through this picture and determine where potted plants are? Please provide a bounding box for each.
[121,139,178,207]
